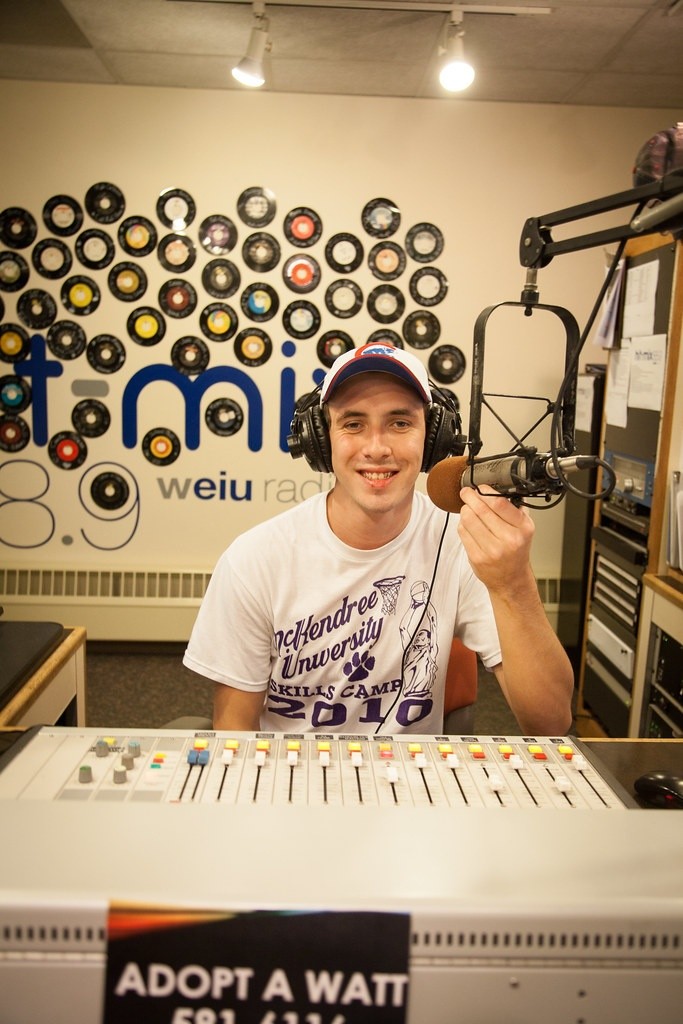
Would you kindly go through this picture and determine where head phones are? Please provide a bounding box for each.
[284,376,467,475]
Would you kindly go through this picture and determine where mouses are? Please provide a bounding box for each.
[634,769,683,810]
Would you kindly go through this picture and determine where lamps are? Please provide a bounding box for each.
[232,1,275,86]
[439,4,476,91]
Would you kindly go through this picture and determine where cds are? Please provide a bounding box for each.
[0,183,466,510]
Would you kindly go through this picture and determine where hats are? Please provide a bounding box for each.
[319,342,433,413]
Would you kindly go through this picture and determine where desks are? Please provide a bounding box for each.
[0,622,88,725]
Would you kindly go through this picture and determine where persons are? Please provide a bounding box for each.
[181,341,574,738]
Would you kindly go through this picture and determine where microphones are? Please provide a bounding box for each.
[427,451,598,513]
